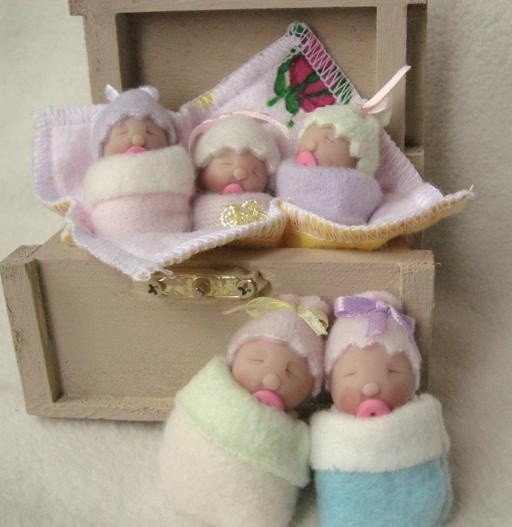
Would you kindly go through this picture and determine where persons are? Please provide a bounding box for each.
[82,84,195,231]
[309,290,453,527]
[195,115,280,229]
[160,294,330,526]
[276,104,392,228]
